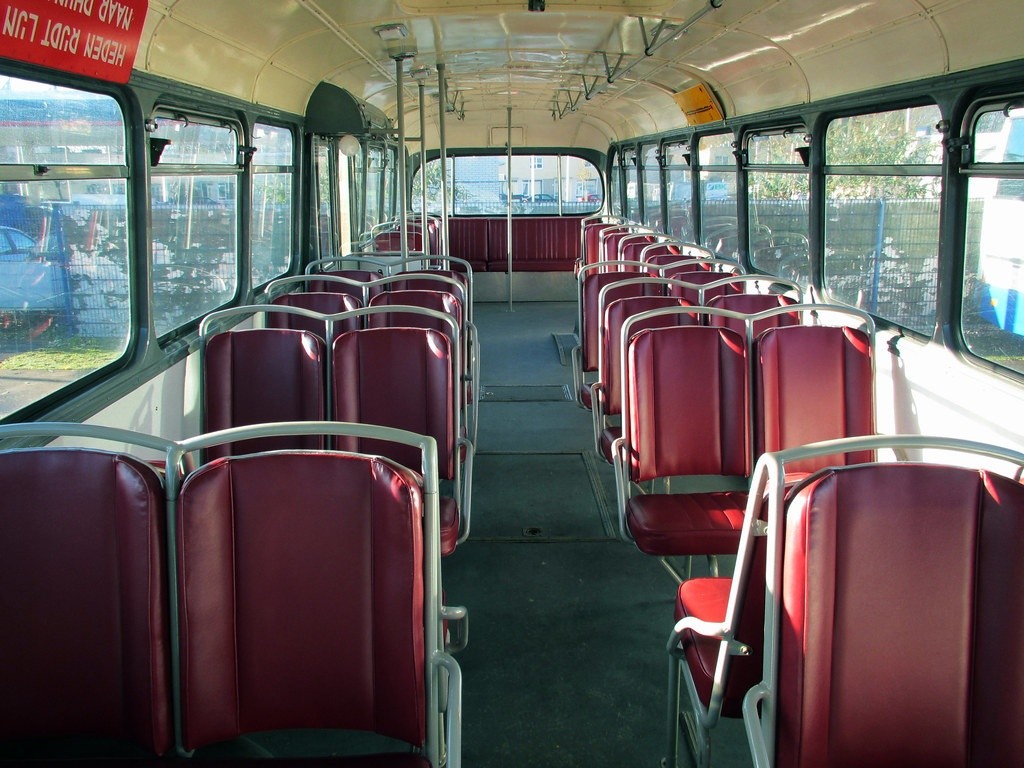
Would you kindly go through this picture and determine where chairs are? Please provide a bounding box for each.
[0,211,482,768]
[575,215,1024,768]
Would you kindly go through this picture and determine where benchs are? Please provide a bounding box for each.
[446,215,599,302]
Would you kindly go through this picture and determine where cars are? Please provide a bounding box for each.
[528,193,557,203]
[0,225,77,319]
[578,193,602,203]
[704,182,729,203]
[183,196,220,207]
[500,193,526,205]
